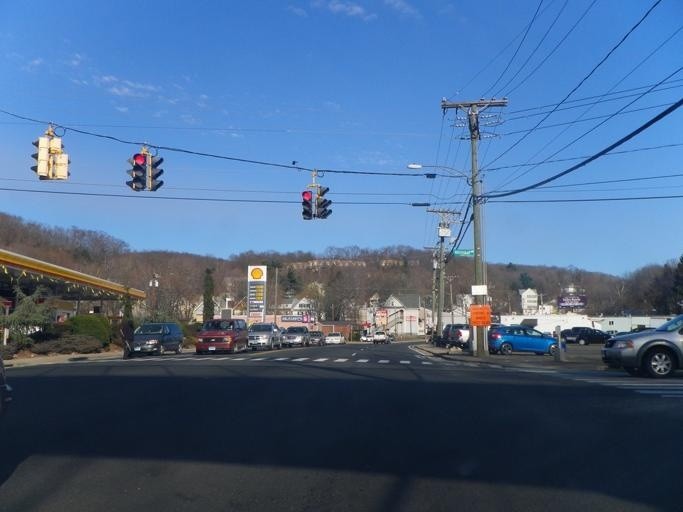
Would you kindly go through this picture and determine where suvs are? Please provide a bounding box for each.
[601,315,683,377]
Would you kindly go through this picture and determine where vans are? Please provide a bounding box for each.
[133,321,183,356]
[196,319,248,353]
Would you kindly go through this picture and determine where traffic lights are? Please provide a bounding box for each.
[149,156,163,191]
[301,190,312,220]
[317,185,329,196]
[47,156,54,180]
[125,157,133,189]
[54,155,70,180]
[30,137,49,177]
[132,153,146,191]
[315,197,332,220]
[52,137,64,155]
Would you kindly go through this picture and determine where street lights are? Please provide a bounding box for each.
[407,163,485,356]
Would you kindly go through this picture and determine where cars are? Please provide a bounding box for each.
[280,326,310,346]
[359,330,393,344]
[308,331,325,346]
[324,331,345,345]
[442,322,655,356]
[246,323,281,351]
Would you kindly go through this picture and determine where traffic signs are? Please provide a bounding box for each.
[453,249,474,256]
